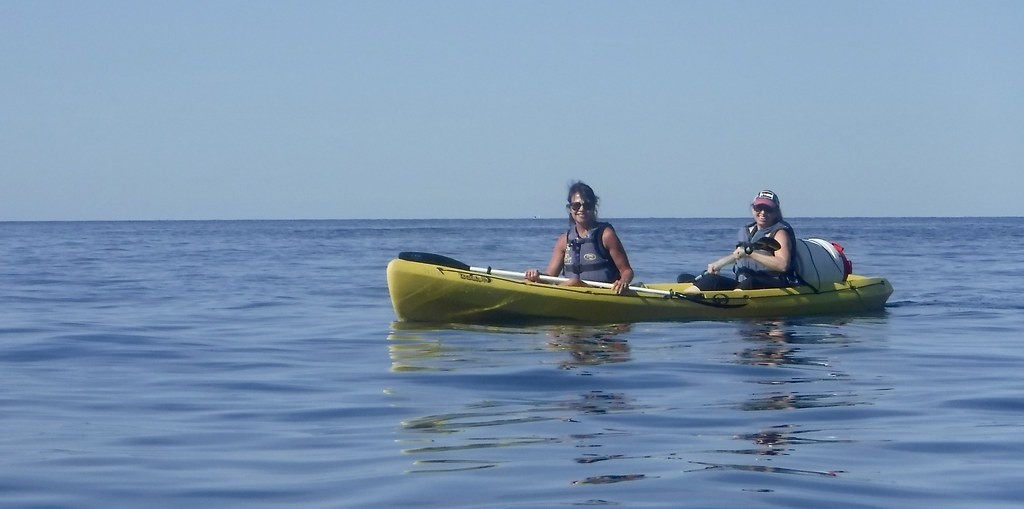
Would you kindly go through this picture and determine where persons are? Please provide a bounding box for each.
[525,183,633,294]
[684,190,796,292]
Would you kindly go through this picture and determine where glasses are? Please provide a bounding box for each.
[752,206,774,213]
[570,201,595,210]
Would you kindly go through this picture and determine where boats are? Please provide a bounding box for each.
[386,259,896,321]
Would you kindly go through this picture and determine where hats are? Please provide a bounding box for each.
[751,190,780,211]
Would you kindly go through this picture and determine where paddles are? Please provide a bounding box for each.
[397,250,752,310]
[688,236,782,282]
